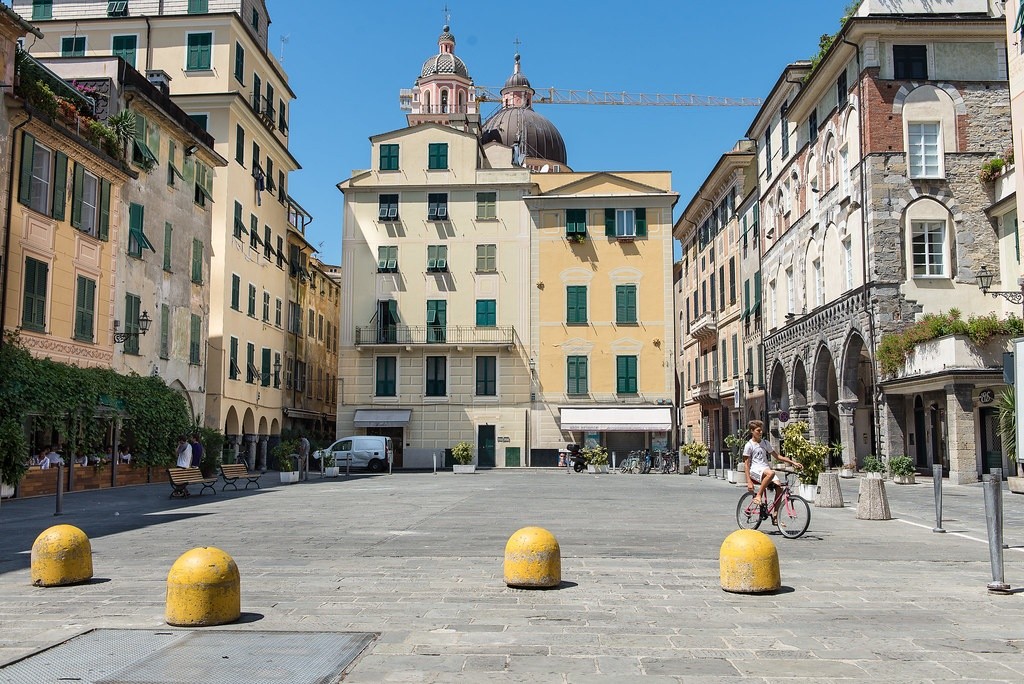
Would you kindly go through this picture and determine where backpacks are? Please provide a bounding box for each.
[200,457,212,479]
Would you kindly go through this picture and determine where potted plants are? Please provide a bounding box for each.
[273,438,301,483]
[684,441,711,476]
[450,442,474,473]
[318,447,340,477]
[579,445,610,473]
[724,419,917,502]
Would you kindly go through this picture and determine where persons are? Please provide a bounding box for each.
[742,420,804,527]
[558,453,567,466]
[25,446,133,469]
[297,433,310,482]
[172,433,203,498]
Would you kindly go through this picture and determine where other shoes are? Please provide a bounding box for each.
[282,454,287,459]
[774,519,786,527]
[298,479,303,482]
[753,497,761,506]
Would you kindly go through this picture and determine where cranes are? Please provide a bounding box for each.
[473,85,762,129]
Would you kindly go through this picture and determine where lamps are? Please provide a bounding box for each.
[767,235,780,239]
[785,313,807,320]
[186,145,198,155]
[745,367,765,390]
[112,309,152,345]
[812,188,827,193]
[257,361,282,381]
[976,264,1024,304]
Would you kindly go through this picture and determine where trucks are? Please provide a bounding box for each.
[311,435,394,475]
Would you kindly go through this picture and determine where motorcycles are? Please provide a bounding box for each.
[566,443,608,473]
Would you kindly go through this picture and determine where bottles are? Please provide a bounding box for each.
[30,456,33,465]
[36,458,40,464]
[32,457,35,465]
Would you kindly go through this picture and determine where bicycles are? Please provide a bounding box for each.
[735,467,811,540]
[620,449,676,475]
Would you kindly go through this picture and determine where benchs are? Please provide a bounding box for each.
[167,466,218,499]
[220,464,261,491]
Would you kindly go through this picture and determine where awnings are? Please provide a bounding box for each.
[353,410,412,427]
[558,405,674,431]
